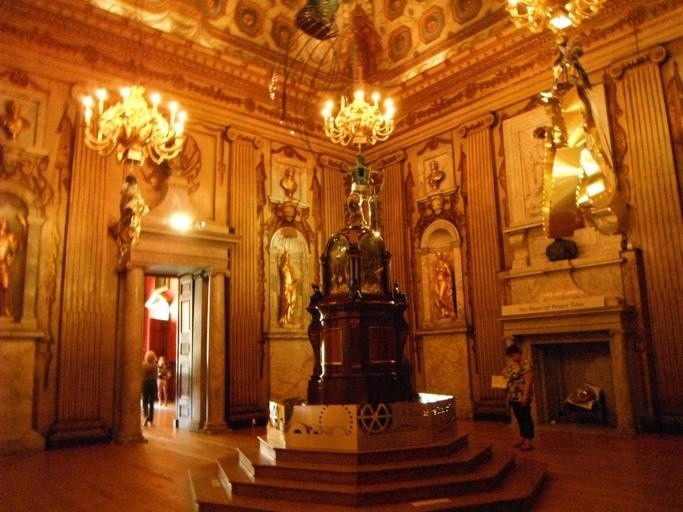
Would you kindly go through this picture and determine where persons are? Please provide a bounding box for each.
[504,344,535,451]
[142,350,159,427]
[0,215,19,317]
[431,250,456,321]
[157,355,172,406]
[277,251,300,327]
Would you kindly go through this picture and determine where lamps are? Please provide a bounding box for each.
[321,66,395,151]
[79,82,189,245]
